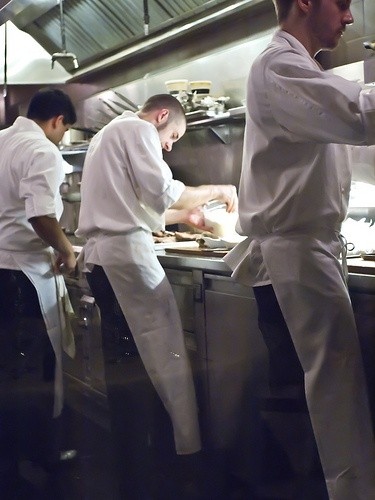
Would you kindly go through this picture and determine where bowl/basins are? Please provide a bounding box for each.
[202,237,225,248]
[361,255,375,260]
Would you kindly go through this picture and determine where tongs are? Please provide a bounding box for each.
[109,90,138,113]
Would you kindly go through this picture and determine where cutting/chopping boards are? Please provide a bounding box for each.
[164,246,231,257]
[340,256,375,275]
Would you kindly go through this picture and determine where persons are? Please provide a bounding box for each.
[222,0,374,500]
[0,88,77,500]
[72,94,240,500]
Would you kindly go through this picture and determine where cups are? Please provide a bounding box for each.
[167,79,188,111]
[190,80,211,111]
